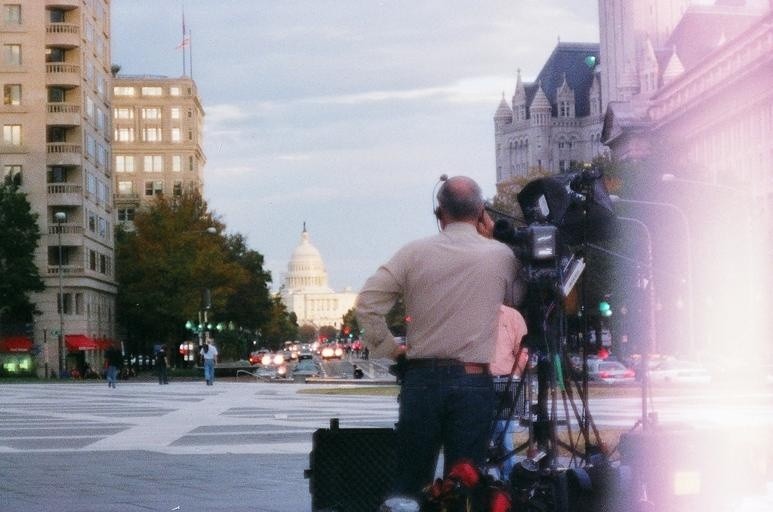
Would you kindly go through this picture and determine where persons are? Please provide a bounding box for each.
[154,346,169,384]
[343,344,370,359]
[352,364,363,378]
[351,175,529,507]
[200,340,218,386]
[103,342,124,388]
[489,304,529,481]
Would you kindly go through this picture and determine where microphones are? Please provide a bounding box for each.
[432,174,448,213]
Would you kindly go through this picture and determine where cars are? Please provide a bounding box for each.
[248,341,343,366]
[587,354,705,385]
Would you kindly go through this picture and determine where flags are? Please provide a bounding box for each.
[175,37,190,50]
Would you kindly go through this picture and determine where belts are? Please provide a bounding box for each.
[492,374,510,378]
[408,358,484,367]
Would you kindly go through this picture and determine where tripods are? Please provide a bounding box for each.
[488,316,605,471]
[606,253,659,461]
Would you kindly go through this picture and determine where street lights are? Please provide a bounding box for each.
[609,173,740,353]
[54,211,66,376]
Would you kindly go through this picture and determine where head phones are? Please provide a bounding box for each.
[434,207,485,233]
